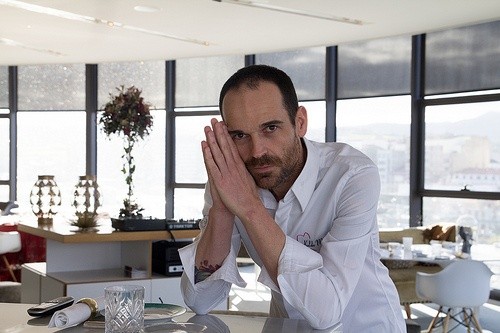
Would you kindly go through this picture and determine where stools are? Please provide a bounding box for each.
[0,231,22,282]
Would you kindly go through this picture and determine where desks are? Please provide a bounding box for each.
[0,302,343,333]
[381,244,472,269]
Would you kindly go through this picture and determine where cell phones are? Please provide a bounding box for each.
[27,296,74,317]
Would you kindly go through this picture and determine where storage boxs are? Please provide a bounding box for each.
[152,240,192,276]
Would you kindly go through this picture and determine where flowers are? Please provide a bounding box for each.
[99,83,154,215]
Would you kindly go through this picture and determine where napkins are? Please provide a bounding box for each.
[47,298,98,329]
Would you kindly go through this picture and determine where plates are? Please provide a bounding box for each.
[99,303,187,320]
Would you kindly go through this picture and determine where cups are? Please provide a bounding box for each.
[387,236,456,257]
[104,284,146,333]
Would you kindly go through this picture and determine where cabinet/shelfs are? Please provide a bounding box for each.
[17,222,231,310]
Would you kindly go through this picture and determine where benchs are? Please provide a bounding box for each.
[380,229,443,319]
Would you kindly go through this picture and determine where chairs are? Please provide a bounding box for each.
[414,260,495,333]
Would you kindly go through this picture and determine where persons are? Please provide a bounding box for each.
[179,64,406,332]
